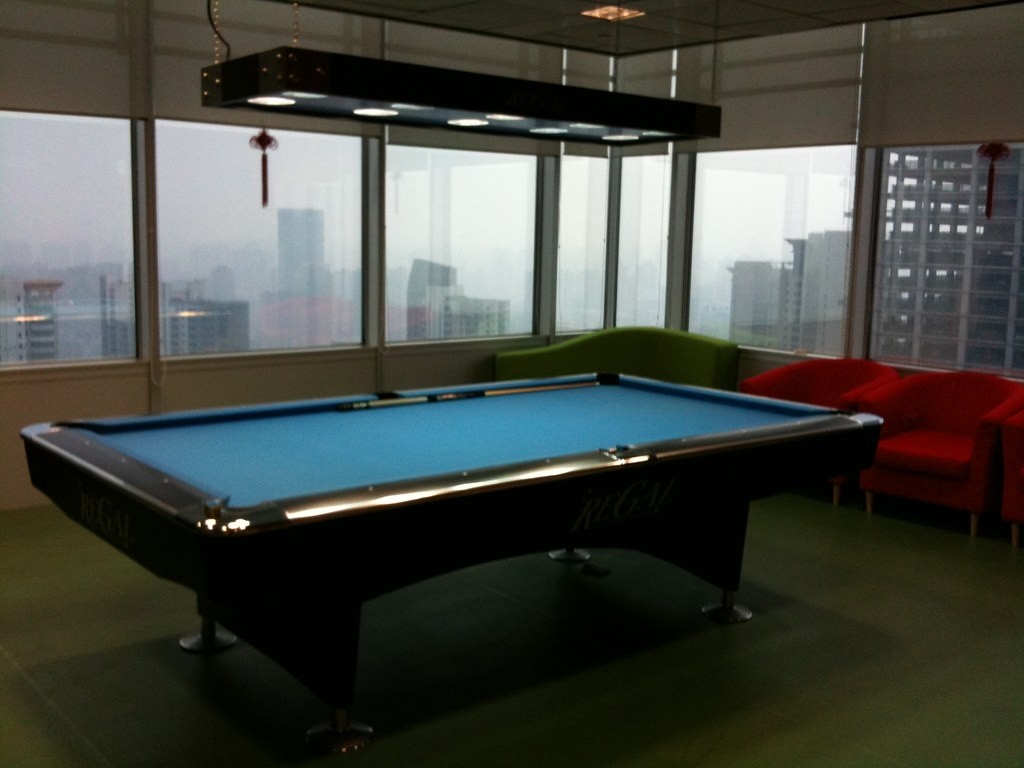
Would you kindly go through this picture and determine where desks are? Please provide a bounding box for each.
[20,371,884,754]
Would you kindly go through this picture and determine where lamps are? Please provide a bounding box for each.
[199,1,723,146]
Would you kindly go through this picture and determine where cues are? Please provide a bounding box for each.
[349,381,600,409]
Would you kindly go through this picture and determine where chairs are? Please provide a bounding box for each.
[740,358,1024,547]
